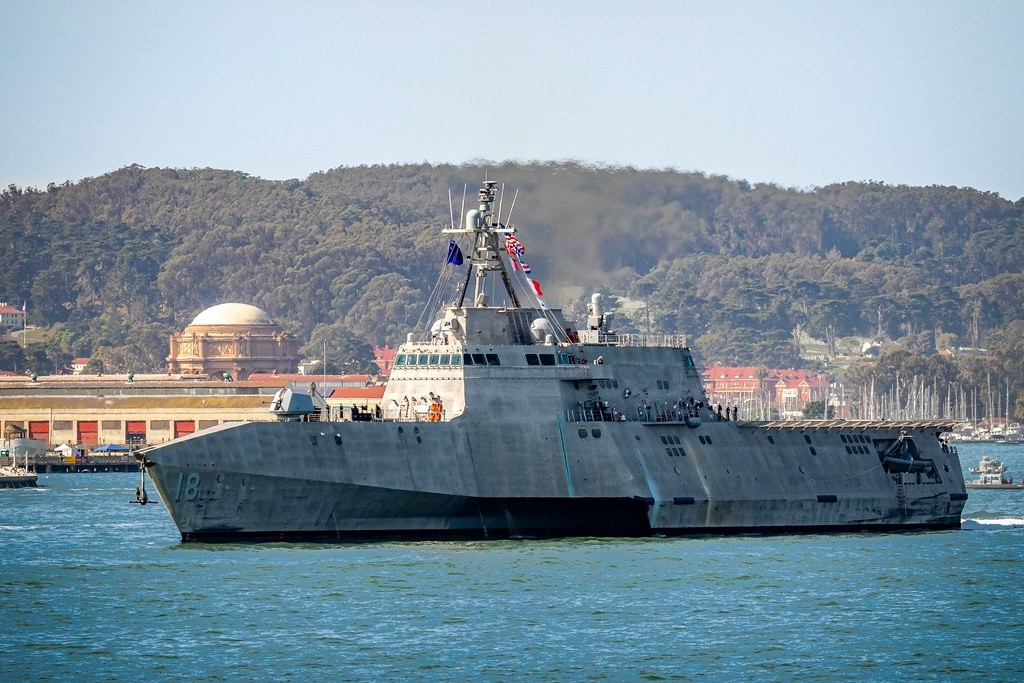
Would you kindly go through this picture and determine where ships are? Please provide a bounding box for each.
[127,182,969,544]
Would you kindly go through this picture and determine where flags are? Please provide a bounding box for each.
[447,239,463,266]
[499,225,549,296]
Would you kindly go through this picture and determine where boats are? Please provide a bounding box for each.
[0,464,38,489]
[963,456,1024,490]
[13,463,147,473]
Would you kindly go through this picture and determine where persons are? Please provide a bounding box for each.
[340,403,343,418]
[376,404,381,418]
[351,403,358,421]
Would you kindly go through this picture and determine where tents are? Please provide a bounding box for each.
[54,443,73,451]
[94,444,129,452]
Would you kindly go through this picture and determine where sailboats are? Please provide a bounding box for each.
[938,373,1024,444]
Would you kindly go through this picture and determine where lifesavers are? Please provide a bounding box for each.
[430,404,441,421]
